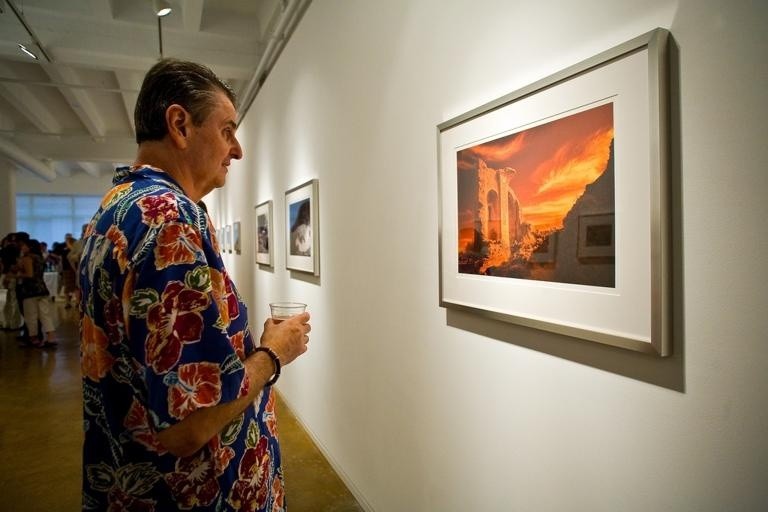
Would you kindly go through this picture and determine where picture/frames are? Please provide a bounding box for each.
[284,178,320,277]
[254,200,274,268]
[435,27,674,360]
[216,222,240,253]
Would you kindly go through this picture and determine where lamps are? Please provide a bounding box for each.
[18,43,38,60]
[152,0,172,17]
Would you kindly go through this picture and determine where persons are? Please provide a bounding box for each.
[0,223,90,350]
[76,57,312,512]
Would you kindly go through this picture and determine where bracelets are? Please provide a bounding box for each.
[251,345,282,387]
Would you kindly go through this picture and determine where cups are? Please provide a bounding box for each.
[269,301,308,327]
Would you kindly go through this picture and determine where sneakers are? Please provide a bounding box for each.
[27,342,59,347]
